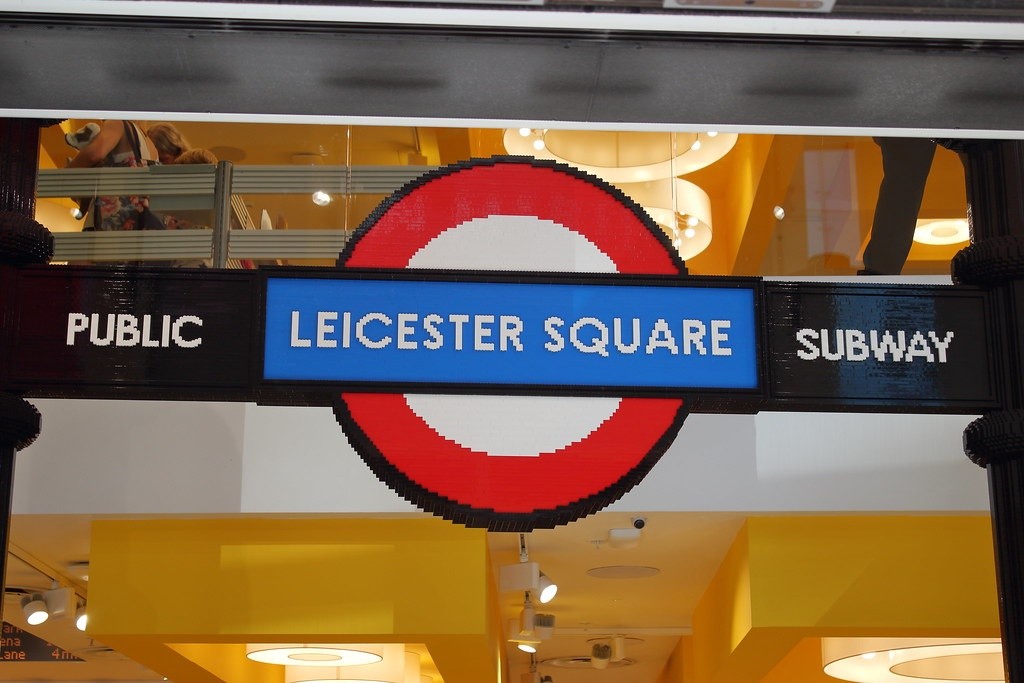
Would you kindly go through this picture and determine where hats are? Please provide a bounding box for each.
[63,122,101,149]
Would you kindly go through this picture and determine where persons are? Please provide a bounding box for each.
[146,123,189,165]
[84,158,164,230]
[175,149,218,164]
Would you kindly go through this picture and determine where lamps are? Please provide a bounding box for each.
[21,592,49,626]
[403,651,420,683]
[821,635,1005,682]
[285,644,406,682]
[516,640,537,653]
[913,219,970,245]
[504,128,738,185]
[619,178,713,261]
[74,599,87,632]
[421,675,433,682]
[246,643,383,667]
[529,569,557,603]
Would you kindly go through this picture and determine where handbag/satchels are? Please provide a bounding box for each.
[134,206,167,231]
